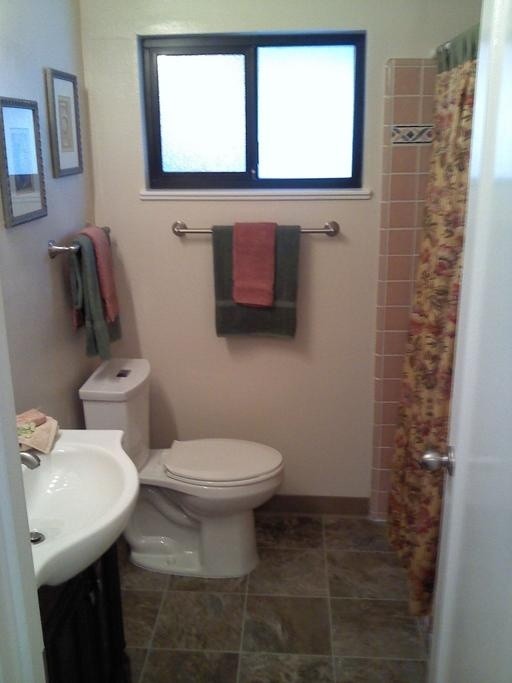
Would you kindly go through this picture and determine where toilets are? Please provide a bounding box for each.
[79,355,284,580]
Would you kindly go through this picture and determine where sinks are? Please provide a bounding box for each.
[22,404,140,586]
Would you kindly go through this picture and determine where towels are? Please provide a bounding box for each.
[211,221,302,338]
[67,226,122,362]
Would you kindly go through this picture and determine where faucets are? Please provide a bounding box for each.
[19,450,42,473]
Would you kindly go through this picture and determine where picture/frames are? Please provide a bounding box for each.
[1,66,85,228]
